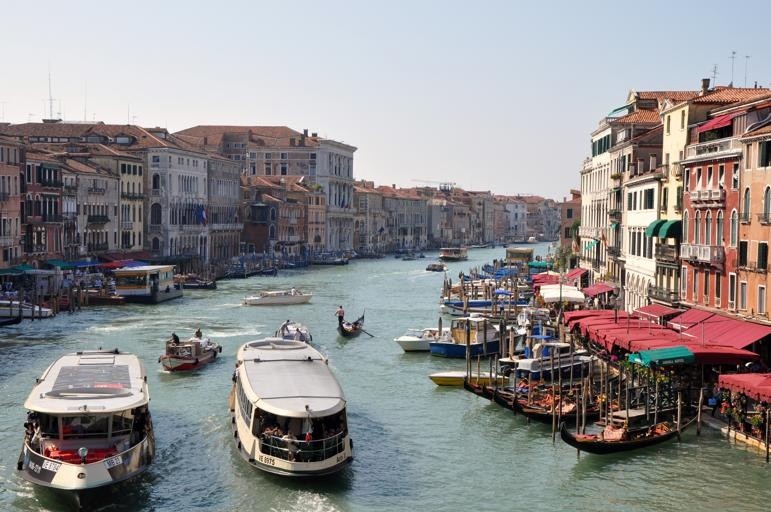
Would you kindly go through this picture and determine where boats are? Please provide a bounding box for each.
[14,349,152,512]
[275,320,312,344]
[158,337,222,372]
[239,287,314,306]
[227,337,355,480]
[337,308,365,337]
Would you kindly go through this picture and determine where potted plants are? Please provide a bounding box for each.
[610,173,622,189]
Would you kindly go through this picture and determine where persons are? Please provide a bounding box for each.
[334,305,345,325]
[293,328,303,341]
[24,411,41,452]
[278,319,290,338]
[497,297,505,314]
[260,426,299,459]
[169,331,179,345]
[194,328,202,339]
[130,405,152,448]
[291,287,297,296]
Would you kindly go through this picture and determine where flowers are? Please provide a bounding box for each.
[597,348,679,385]
[720,390,769,441]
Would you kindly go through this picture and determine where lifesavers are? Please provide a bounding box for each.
[78,447,88,457]
